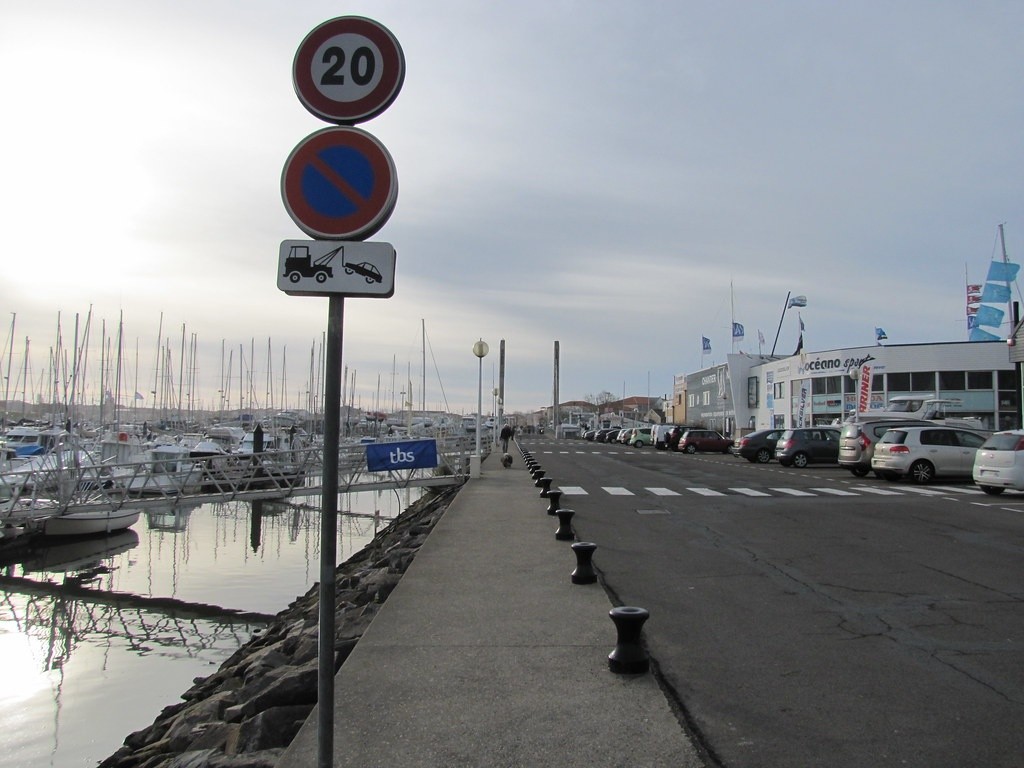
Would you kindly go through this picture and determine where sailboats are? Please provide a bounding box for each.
[0,304,497,540]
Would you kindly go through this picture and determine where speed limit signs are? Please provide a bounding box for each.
[292,16,407,121]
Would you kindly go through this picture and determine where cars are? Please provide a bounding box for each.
[615,429,627,443]
[630,428,652,449]
[972,429,1024,494]
[732,429,789,464]
[605,429,621,445]
[678,430,735,454]
[583,429,596,441]
[620,428,632,446]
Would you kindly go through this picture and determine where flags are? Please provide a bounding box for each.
[970,327,1000,344]
[982,283,1011,303]
[701,335,712,355]
[135,392,145,400]
[405,401,414,407]
[797,382,808,431]
[966,283,982,329]
[792,313,806,356]
[985,260,1020,281]
[859,362,874,412]
[758,332,766,344]
[876,327,887,340]
[788,295,807,308]
[732,322,744,342]
[974,305,1004,328]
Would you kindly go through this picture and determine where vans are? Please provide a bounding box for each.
[650,423,680,450]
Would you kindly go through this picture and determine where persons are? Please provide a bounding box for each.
[500,423,531,453]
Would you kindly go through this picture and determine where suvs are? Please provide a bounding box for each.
[870,427,987,486]
[838,416,941,478]
[774,427,842,468]
[664,426,708,452]
[593,428,613,443]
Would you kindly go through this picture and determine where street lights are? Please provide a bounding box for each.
[848,368,860,423]
[491,388,497,443]
[471,337,489,457]
